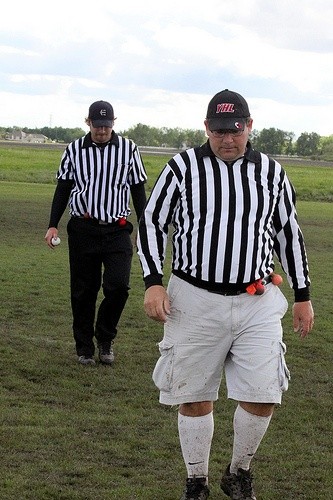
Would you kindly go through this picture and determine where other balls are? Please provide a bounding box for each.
[52,237,61,245]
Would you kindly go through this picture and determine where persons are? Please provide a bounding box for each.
[134,88,315,500]
[44,100,150,364]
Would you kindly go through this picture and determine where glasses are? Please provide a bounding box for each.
[211,130,244,137]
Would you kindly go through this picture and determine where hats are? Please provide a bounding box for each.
[89,100,114,127]
[205,89,251,133]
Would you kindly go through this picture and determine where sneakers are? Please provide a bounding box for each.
[181,477,210,500]
[97,339,115,364]
[79,355,96,366]
[220,463,256,500]
